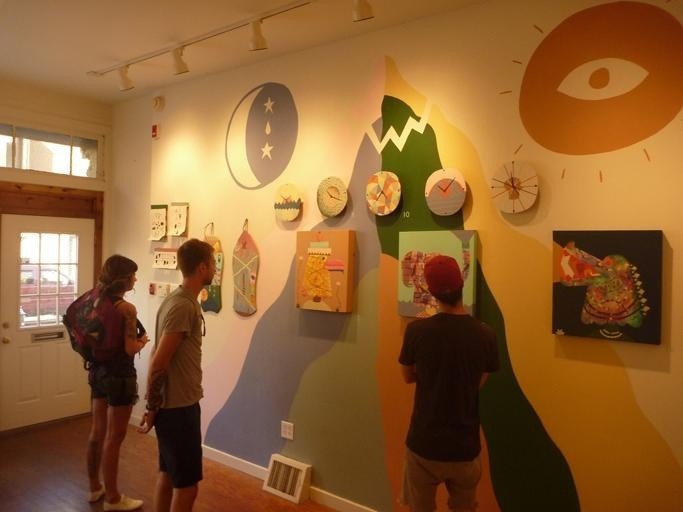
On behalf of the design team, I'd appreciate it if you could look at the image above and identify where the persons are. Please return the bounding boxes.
[398,255,499,512]
[136,238,217,512]
[62,254,151,511]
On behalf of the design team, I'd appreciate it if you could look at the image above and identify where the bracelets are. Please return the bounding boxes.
[146,403,160,411]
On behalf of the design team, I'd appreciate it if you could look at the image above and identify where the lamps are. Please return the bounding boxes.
[84,1,374,93]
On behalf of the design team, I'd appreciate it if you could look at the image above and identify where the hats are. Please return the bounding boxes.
[423,255,464,294]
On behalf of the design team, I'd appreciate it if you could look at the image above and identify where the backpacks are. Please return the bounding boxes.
[63,285,124,367]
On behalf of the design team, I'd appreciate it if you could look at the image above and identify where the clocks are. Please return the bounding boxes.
[270,159,540,225]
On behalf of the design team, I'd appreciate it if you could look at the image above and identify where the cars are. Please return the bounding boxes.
[20,264,76,317]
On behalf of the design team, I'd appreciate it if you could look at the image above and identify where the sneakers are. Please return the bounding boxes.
[87,480,106,502]
[102,493,144,511]
